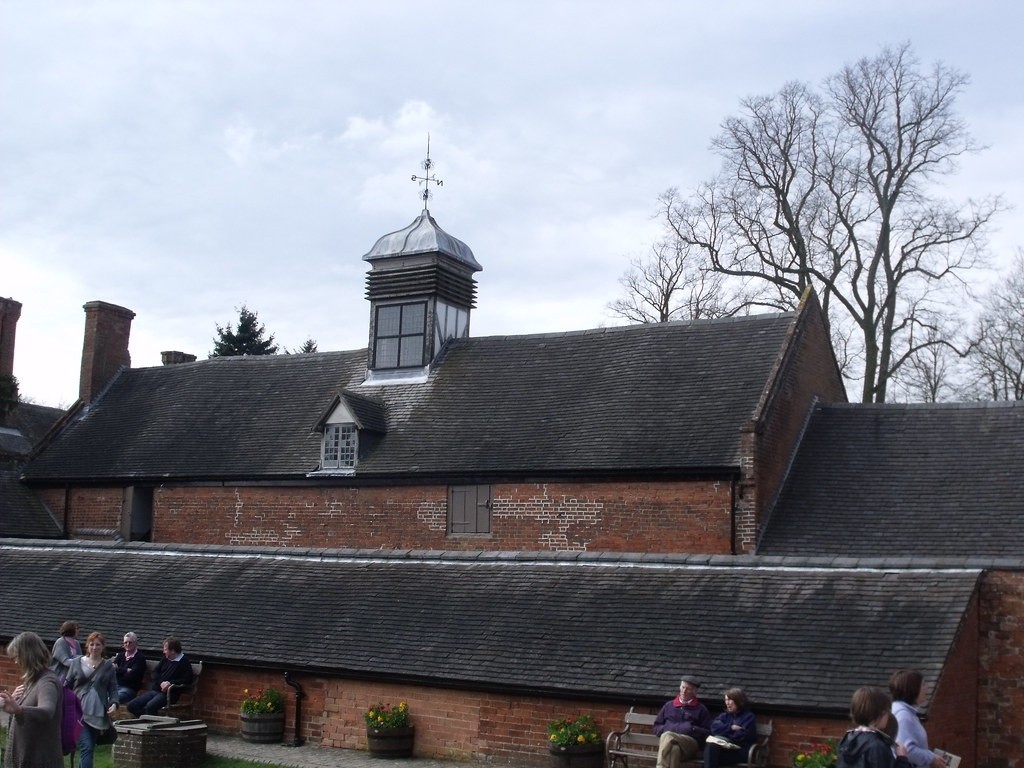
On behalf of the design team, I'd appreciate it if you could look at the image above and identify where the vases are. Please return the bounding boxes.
[366,730,414,760]
[550,742,605,768]
[242,715,286,745]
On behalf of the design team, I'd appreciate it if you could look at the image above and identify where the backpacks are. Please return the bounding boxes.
[37,673,84,756]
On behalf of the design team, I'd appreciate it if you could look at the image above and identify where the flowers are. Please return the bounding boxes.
[791,738,837,768]
[546,713,598,744]
[240,688,286,716]
[363,699,409,727]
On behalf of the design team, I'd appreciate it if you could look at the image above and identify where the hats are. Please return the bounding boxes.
[680,676,701,689]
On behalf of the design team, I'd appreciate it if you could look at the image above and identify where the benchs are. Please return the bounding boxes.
[606,707,773,768]
[146,659,203,719]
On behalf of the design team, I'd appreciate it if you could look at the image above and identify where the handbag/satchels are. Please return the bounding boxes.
[706,736,742,749]
[94,725,118,745]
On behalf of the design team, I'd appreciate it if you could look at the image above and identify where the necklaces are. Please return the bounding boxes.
[86,657,102,669]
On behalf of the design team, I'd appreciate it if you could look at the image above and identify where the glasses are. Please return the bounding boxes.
[123,642,133,644]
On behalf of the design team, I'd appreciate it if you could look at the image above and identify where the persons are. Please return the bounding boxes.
[51,619,83,688]
[652,675,713,768]
[837,685,912,768]
[888,670,950,768]
[703,686,758,768]
[0,632,65,768]
[64,631,120,768]
[127,637,193,718]
[113,631,147,704]
